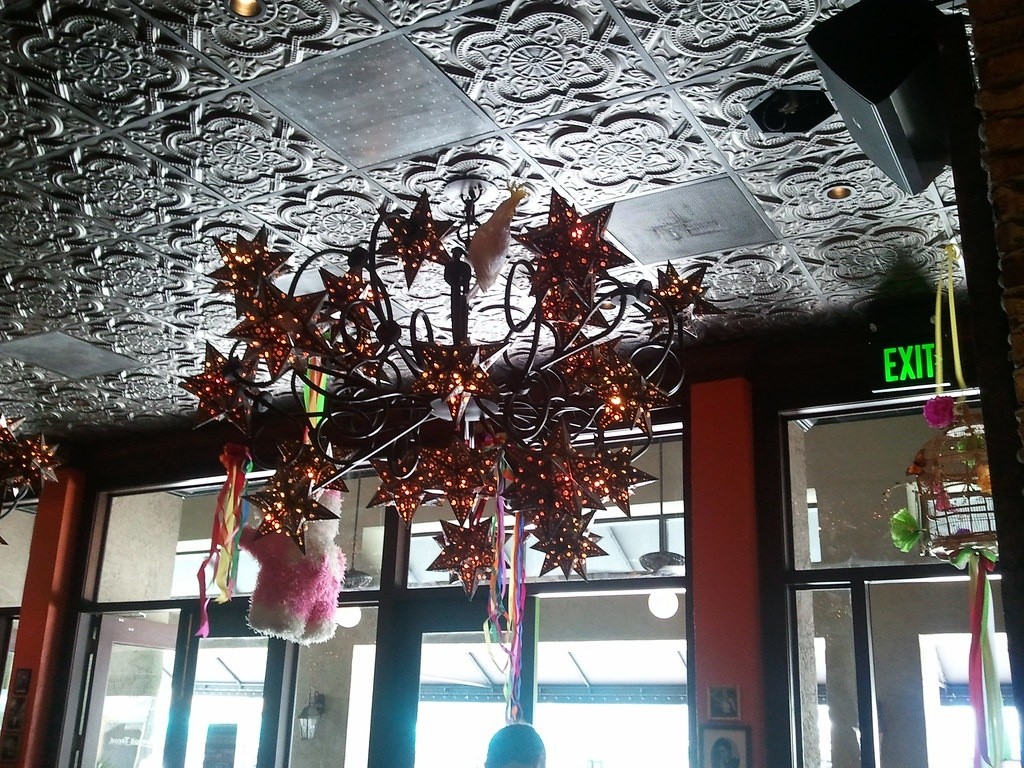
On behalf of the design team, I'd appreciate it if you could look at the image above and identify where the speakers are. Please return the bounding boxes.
[804,0,979,197]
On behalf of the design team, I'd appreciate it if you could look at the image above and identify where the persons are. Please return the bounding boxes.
[484,723,547,768]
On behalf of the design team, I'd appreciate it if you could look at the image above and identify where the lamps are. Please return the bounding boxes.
[181,174,729,601]
[0,413,63,519]
[297,686,324,741]
[639,441,686,618]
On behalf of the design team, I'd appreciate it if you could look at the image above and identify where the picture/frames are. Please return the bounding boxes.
[8,695,29,733]
[706,683,742,721]
[13,668,32,693]
[1,735,21,763]
[697,724,753,768]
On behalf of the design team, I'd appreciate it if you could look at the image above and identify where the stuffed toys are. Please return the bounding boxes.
[232,487,346,646]
